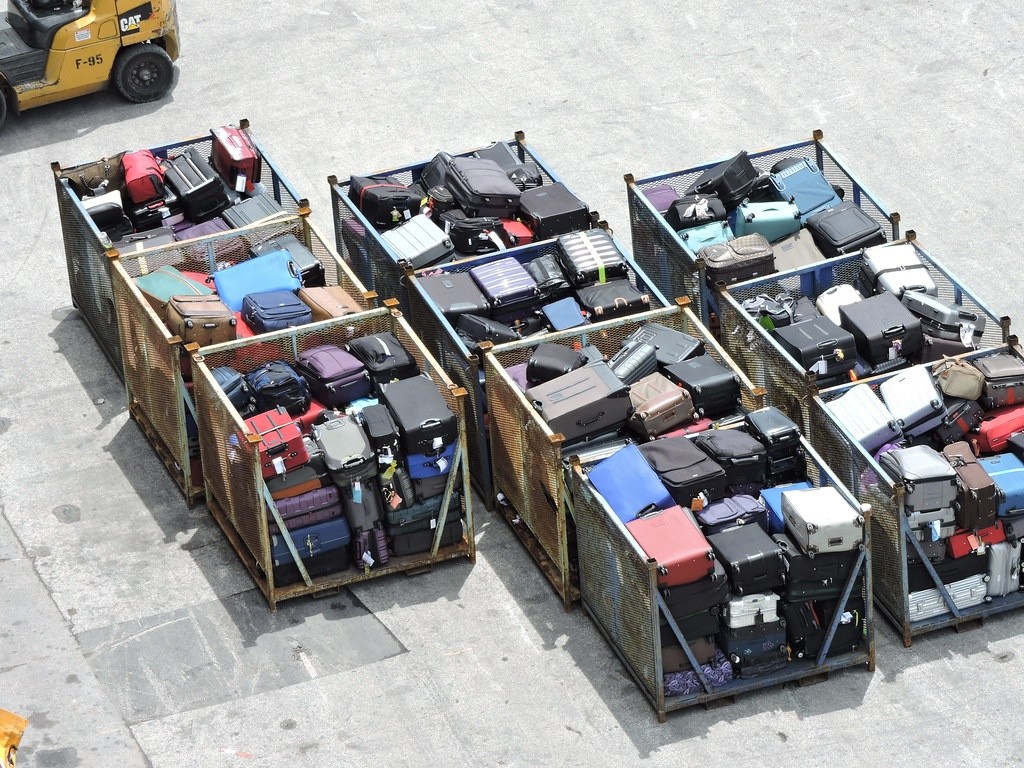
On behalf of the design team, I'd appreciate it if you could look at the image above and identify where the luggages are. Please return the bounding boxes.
[63,117,1024,702]
